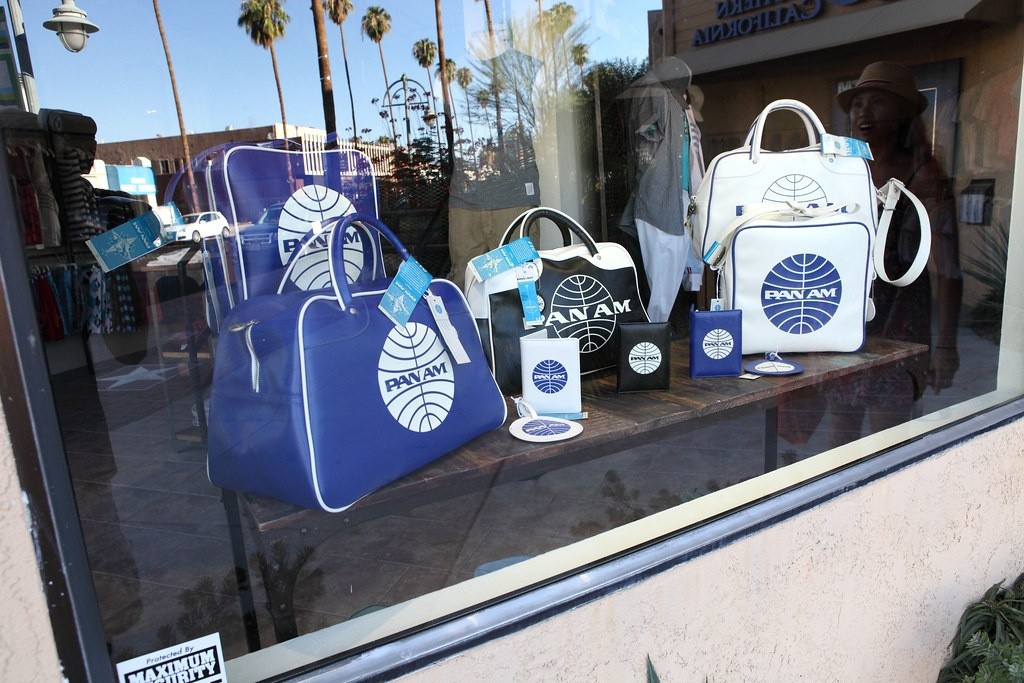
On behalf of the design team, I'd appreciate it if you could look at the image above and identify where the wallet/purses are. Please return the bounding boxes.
[616,321,671,394]
[688,303,743,378]
[519,329,583,413]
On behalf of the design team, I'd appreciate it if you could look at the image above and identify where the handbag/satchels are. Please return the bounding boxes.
[464,206,655,395]
[684,99,881,279]
[703,199,873,354]
[163,130,388,338]
[204,215,508,518]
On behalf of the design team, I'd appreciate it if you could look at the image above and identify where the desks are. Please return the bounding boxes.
[222,329,931,653]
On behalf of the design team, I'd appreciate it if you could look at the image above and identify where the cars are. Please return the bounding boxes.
[165,212,231,243]
[235,203,285,246]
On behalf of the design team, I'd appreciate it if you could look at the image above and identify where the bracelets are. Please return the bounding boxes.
[935,346,958,350]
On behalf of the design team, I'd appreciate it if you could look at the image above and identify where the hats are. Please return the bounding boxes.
[686,84,704,122]
[651,56,690,110]
[834,61,929,117]
[680,59,692,105]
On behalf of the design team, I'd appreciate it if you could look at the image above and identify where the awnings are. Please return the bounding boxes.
[628,0,1017,90]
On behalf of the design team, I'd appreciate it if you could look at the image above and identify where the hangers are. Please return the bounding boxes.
[25,245,189,276]
[3,131,129,157]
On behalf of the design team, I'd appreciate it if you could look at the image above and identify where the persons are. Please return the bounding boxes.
[432,28,547,292]
[825,59,963,451]
[618,55,712,329]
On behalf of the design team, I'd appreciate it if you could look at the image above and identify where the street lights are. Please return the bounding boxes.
[8,1,102,117]
